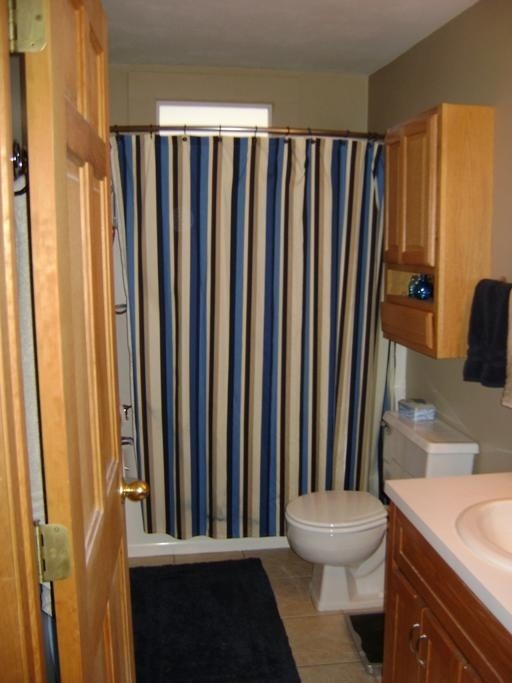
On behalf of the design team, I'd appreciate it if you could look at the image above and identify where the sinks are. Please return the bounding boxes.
[454,496,511,568]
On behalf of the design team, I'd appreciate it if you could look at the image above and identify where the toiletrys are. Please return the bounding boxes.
[408,275,418,297]
[415,273,432,300]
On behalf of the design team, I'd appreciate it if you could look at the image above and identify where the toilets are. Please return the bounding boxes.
[283,409,481,615]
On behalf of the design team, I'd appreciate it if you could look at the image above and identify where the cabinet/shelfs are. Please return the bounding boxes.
[381,478,512,682]
[378,102,498,361]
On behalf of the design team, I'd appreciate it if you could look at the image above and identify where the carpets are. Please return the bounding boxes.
[350,611,388,664]
[129,557,302,681]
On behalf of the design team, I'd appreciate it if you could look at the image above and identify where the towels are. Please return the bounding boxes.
[500,291,512,409]
[462,278,511,388]
